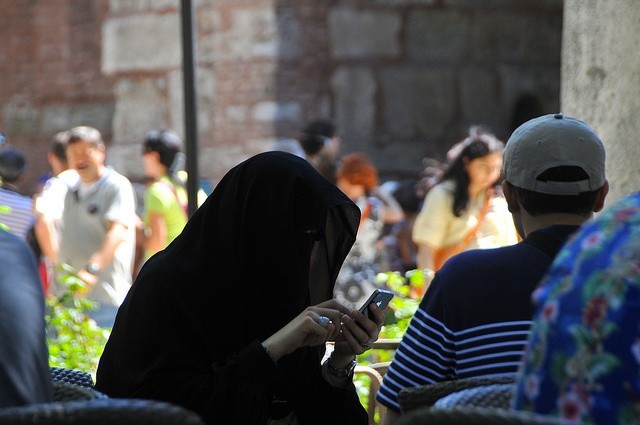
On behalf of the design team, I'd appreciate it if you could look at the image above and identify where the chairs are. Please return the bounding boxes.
[351,355,398,424]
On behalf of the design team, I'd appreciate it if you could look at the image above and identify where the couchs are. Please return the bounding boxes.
[399,189,640,424]
[0,228,200,424]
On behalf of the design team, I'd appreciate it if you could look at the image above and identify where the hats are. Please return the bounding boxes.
[503,112,606,196]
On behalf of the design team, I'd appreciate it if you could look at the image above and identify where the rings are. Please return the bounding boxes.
[361,337,373,346]
[319,315,330,329]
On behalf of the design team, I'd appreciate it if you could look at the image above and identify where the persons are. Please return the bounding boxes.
[373,111,609,425]
[35,125,78,230]
[95,148,387,425]
[294,115,342,175]
[2,144,54,264]
[509,188,640,424]
[45,125,135,344]
[328,149,406,312]
[132,213,146,249]
[133,126,209,279]
[410,132,505,289]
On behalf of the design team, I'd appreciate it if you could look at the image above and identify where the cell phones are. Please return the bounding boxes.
[352,288,394,323]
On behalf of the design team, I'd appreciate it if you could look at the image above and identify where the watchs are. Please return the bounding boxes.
[329,350,358,378]
[82,261,106,278]
